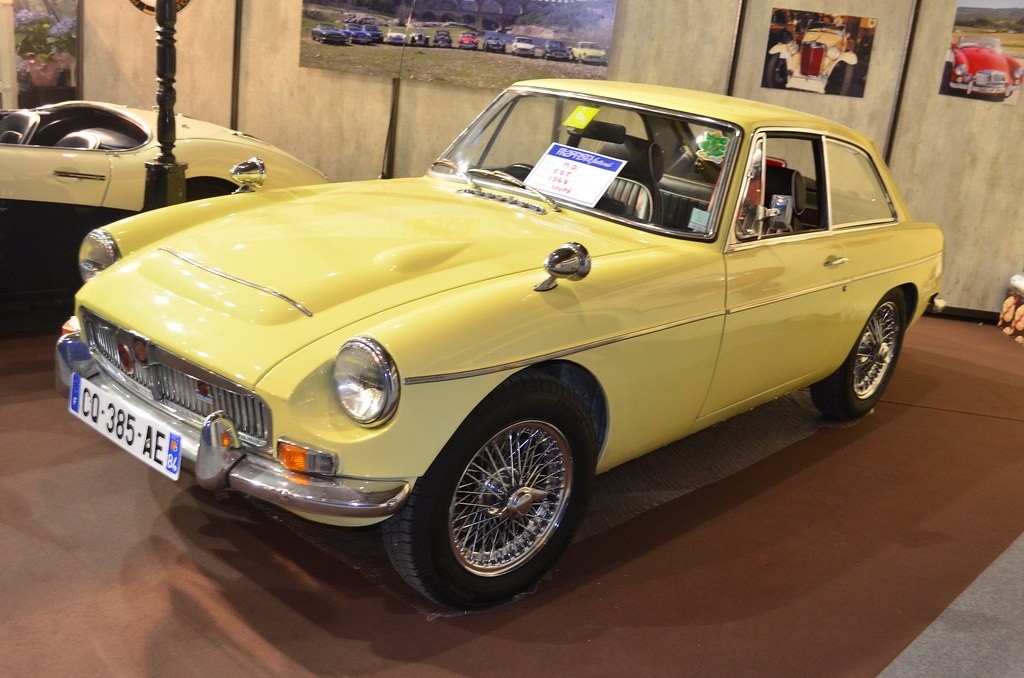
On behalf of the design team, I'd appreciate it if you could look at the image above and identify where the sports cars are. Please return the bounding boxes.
[947,35,1024,102]
[0,100,330,241]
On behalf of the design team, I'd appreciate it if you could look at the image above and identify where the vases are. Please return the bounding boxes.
[30,64,60,86]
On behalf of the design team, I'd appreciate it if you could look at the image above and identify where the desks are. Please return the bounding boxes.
[17,85,76,109]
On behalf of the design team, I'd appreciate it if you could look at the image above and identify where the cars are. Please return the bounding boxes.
[56,79,944,610]
[311,23,606,64]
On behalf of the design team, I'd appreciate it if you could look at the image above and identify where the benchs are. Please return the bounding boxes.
[659,174,715,230]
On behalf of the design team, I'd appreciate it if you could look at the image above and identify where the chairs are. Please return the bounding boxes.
[595,135,664,225]
[0,109,41,145]
[765,164,807,232]
[54,132,101,150]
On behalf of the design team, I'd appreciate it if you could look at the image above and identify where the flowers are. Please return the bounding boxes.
[14,8,79,73]
